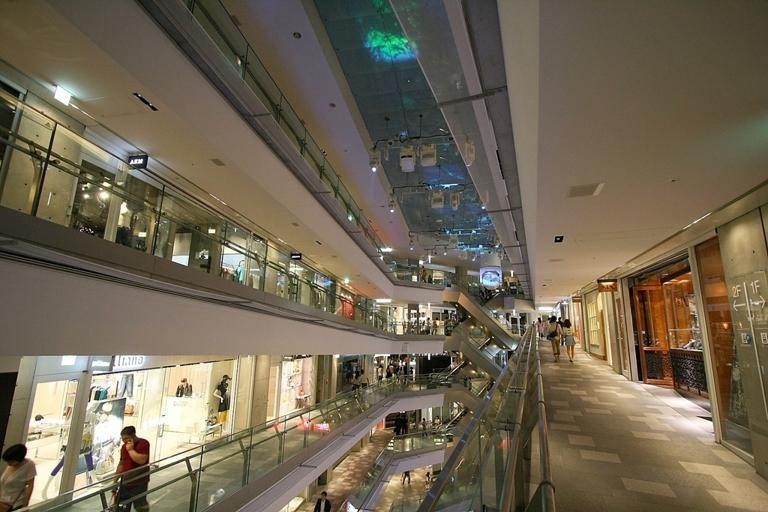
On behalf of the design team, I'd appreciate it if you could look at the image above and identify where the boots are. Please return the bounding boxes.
[552,355,559,362]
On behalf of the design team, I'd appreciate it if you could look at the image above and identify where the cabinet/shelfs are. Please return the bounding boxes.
[26,416,69,458]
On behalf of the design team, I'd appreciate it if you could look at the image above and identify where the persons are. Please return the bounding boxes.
[402,415,408,433]
[110,427,151,512]
[562,319,577,364]
[175,378,194,397]
[400,468,413,483]
[1,442,35,511]
[433,416,440,429]
[547,316,563,362]
[535,315,563,340]
[116,201,134,246]
[127,211,146,247]
[213,374,232,431]
[421,416,429,439]
[424,314,455,336]
[479,286,493,303]
[395,415,402,434]
[350,361,411,391]
[314,491,331,512]
[428,274,433,283]
[420,264,428,283]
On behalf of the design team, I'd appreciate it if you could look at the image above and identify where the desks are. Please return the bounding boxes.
[179,424,223,450]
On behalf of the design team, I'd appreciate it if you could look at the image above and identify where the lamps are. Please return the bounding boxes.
[368,115,502,257]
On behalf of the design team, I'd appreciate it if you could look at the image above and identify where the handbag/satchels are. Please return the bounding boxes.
[100,493,119,512]
[548,331,558,338]
